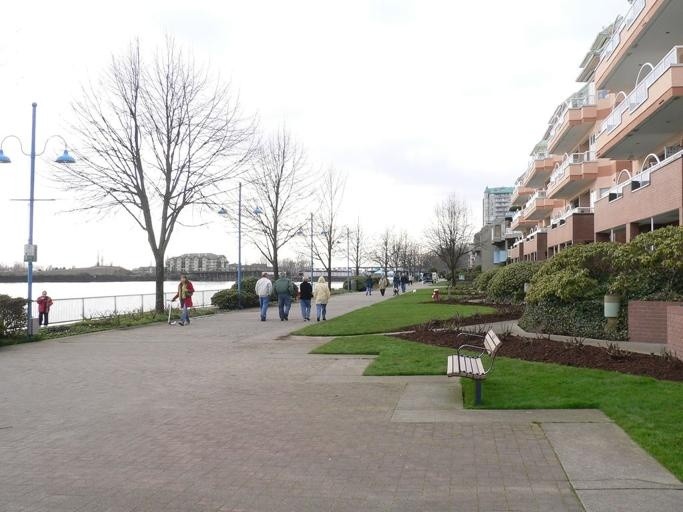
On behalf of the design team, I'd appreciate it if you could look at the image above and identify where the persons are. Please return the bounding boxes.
[272,273,294,321]
[254,273,273,321]
[379,275,388,296]
[393,270,414,293]
[314,276,330,321]
[364,275,373,296]
[37,291,53,328]
[300,276,314,322]
[170,275,195,326]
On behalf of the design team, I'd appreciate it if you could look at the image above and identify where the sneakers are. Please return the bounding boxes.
[178,320,190,326]
[281,317,288,321]
[304,318,310,321]
[261,318,266,321]
[317,319,326,321]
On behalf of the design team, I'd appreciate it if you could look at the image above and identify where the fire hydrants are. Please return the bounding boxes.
[431,288,441,303]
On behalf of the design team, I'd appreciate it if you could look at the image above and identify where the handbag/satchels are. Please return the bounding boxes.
[185,291,192,296]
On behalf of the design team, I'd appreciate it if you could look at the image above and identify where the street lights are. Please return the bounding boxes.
[217,182,264,309]
[0,102,76,337]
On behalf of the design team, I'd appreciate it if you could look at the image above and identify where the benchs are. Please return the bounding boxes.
[446,328,503,404]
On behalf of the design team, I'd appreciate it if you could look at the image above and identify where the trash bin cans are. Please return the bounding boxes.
[351,280,358,291]
